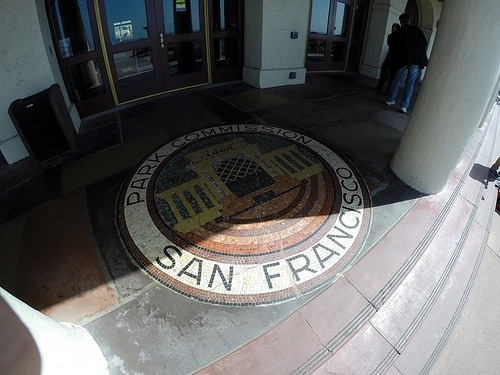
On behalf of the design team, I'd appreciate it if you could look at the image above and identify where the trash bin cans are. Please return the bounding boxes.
[6,83,80,167]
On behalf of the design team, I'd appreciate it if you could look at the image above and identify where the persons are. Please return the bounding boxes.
[385,12,429,114]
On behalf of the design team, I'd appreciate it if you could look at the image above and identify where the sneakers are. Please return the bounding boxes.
[386,100,395,105]
[400,107,407,114]
